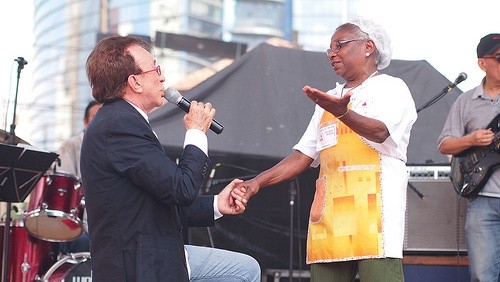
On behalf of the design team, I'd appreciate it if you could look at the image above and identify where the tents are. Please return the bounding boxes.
[148,41,464,282]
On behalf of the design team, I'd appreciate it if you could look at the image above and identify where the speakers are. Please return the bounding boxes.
[404,163,469,255]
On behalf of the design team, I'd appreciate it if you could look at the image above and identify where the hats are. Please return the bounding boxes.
[336,20,392,70]
[477,34,500,58]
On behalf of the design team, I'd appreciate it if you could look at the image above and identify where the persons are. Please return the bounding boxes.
[54,100,103,254]
[229,20,419,282]
[80,35,261,282]
[437,32,500,282]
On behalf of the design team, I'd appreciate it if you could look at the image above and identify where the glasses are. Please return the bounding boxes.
[142,65,161,75]
[483,53,500,63]
[327,37,367,60]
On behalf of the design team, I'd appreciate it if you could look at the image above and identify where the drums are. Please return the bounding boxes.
[0,218,60,282]
[38,251,93,282]
[24,170,84,243]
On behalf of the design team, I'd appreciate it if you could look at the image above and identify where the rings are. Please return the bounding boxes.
[316,99,318,102]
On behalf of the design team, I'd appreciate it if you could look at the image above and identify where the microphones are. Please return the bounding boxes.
[164,87,224,135]
[205,166,216,194]
[453,72,467,87]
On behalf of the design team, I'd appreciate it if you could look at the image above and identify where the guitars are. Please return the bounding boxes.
[450,111,500,198]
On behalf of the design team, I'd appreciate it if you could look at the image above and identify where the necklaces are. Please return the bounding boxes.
[341,70,376,97]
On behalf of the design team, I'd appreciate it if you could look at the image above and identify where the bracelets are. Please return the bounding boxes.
[336,109,349,119]
